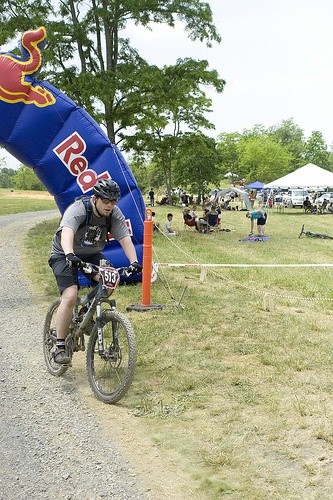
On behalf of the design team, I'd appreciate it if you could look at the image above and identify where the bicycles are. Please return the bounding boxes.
[43,260,140,405]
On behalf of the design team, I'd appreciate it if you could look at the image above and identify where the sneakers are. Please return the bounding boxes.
[85,319,96,336]
[53,347,71,364]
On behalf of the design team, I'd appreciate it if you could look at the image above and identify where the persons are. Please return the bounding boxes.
[48,179,143,364]
[163,213,178,236]
[147,187,154,207]
[152,212,160,232]
[250,191,256,208]
[303,192,333,215]
[220,191,238,211]
[246,209,267,237]
[164,186,217,206]
[183,204,221,234]
[262,192,272,208]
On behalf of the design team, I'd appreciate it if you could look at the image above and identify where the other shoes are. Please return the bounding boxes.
[257,234,264,237]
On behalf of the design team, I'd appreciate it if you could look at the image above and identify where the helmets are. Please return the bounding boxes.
[94,178,121,201]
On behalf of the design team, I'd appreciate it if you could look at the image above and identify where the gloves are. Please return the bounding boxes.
[130,261,142,274]
[66,253,81,268]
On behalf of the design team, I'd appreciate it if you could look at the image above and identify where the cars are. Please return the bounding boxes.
[309,188,333,207]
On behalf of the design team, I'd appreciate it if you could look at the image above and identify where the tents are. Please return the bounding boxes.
[244,181,271,191]
[217,187,252,210]
[263,163,333,214]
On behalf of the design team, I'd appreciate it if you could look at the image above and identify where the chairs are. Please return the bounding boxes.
[199,213,220,233]
[303,203,311,212]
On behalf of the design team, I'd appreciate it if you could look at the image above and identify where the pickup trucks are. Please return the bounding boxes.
[282,189,311,208]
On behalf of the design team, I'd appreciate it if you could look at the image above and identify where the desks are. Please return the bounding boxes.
[229,202,241,210]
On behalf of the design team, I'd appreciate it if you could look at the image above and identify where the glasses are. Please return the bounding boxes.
[100,196,116,205]
